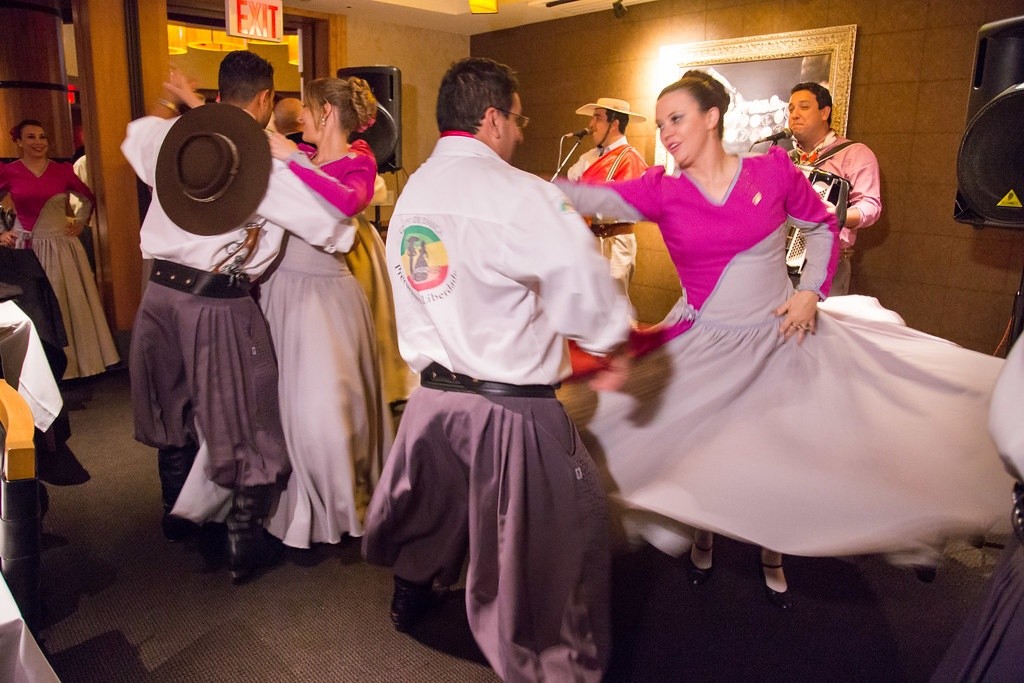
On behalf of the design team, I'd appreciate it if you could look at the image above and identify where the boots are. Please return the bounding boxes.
[157,442,197,543]
[223,482,274,584]
[390,574,425,630]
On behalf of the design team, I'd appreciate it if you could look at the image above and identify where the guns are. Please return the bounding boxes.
[213,221,262,274]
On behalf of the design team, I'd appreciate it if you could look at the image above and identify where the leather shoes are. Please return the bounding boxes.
[685,536,715,586]
[758,554,790,598]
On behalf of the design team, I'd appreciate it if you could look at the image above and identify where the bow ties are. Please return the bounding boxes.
[801,152,818,163]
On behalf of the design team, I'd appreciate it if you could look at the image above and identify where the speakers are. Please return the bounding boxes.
[337,65,403,174]
[953,15,1024,230]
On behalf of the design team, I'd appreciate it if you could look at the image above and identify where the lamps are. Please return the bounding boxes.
[186,28,249,51]
[287,34,299,65]
[247,35,289,46]
[166,23,188,56]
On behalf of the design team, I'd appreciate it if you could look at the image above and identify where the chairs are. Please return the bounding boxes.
[0,379,41,625]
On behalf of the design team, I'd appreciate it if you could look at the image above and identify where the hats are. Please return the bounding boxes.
[575,97,647,122]
[155,103,271,235]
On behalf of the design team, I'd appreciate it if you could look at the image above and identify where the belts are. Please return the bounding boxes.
[420,363,561,398]
[590,222,635,240]
[148,258,253,299]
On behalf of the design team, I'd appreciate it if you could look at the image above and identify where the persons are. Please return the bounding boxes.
[545,71,1016,613]
[933,330,1024,683]
[123,51,419,584]
[363,56,639,683]
[0,118,122,486]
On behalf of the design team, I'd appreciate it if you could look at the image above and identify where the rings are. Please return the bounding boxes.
[799,322,810,331]
[791,322,800,329]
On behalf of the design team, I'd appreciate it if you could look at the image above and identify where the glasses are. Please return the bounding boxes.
[481,106,529,130]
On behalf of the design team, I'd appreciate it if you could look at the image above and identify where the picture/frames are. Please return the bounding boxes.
[653,23,859,175]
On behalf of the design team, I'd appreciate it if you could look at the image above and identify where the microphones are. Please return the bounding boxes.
[565,128,593,138]
[754,129,793,144]
